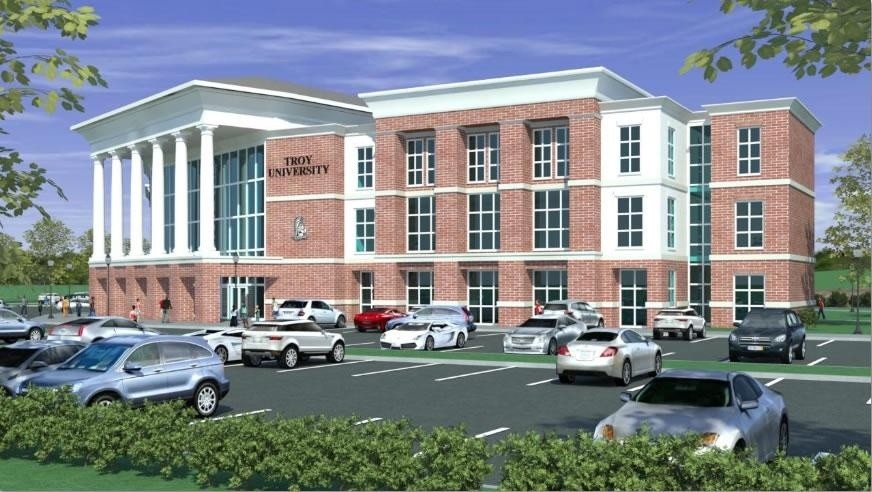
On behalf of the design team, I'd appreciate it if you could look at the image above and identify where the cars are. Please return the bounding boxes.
[592,368,790,465]
[728,308,806,364]
[182,298,477,368]
[503,299,707,386]
[0,291,230,418]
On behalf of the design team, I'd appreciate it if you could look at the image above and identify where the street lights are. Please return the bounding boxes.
[232,252,239,326]
[47,253,111,319]
